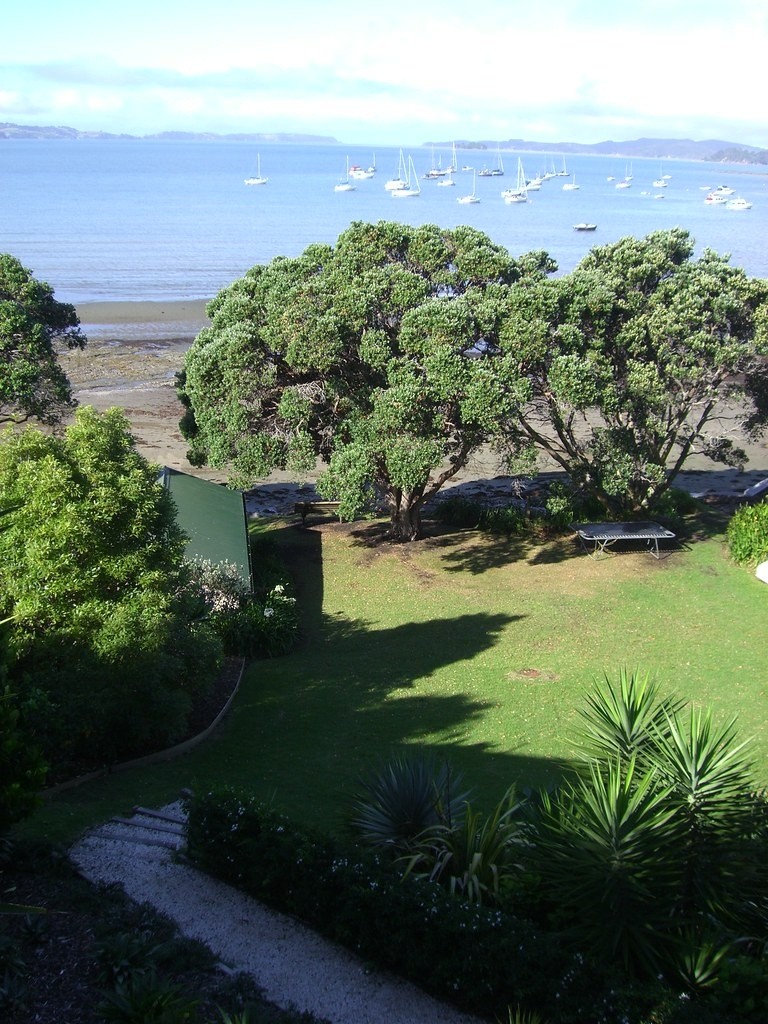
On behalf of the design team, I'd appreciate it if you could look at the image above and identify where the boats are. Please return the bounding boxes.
[725,198,752,210]
[713,185,737,195]
[573,223,598,231]
[478,168,492,176]
[699,185,712,191]
[704,193,727,205]
[461,165,474,171]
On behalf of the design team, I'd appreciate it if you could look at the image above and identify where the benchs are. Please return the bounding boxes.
[295,501,342,524]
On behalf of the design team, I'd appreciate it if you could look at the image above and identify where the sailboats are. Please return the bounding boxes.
[333,154,357,192]
[500,150,569,205]
[383,148,408,190]
[608,157,671,200]
[491,145,504,176]
[421,140,458,179]
[437,164,457,187]
[349,150,378,181]
[243,152,270,185]
[563,172,580,190]
[392,154,421,197]
[456,169,482,204]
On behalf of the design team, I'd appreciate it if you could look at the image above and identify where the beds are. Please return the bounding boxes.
[567,521,676,561]
